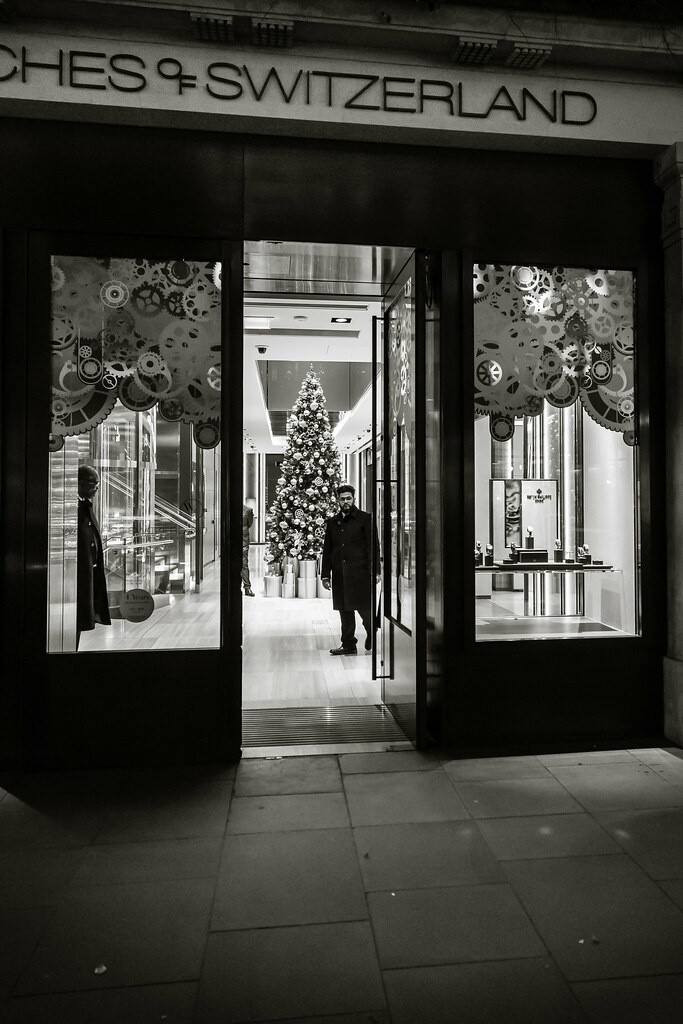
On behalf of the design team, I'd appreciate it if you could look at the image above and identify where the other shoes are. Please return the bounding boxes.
[328,646,357,655]
[245,590,255,597]
[364,633,371,650]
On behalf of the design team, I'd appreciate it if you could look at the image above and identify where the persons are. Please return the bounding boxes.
[320,486,380,655]
[76,465,110,649]
[242,505,254,597]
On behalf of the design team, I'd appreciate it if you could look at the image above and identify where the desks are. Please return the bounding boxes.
[475,566,618,617]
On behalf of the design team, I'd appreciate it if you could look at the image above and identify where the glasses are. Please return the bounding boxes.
[89,481,101,487]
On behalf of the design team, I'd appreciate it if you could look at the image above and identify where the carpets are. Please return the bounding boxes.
[241,703,408,748]
[386,703,417,747]
